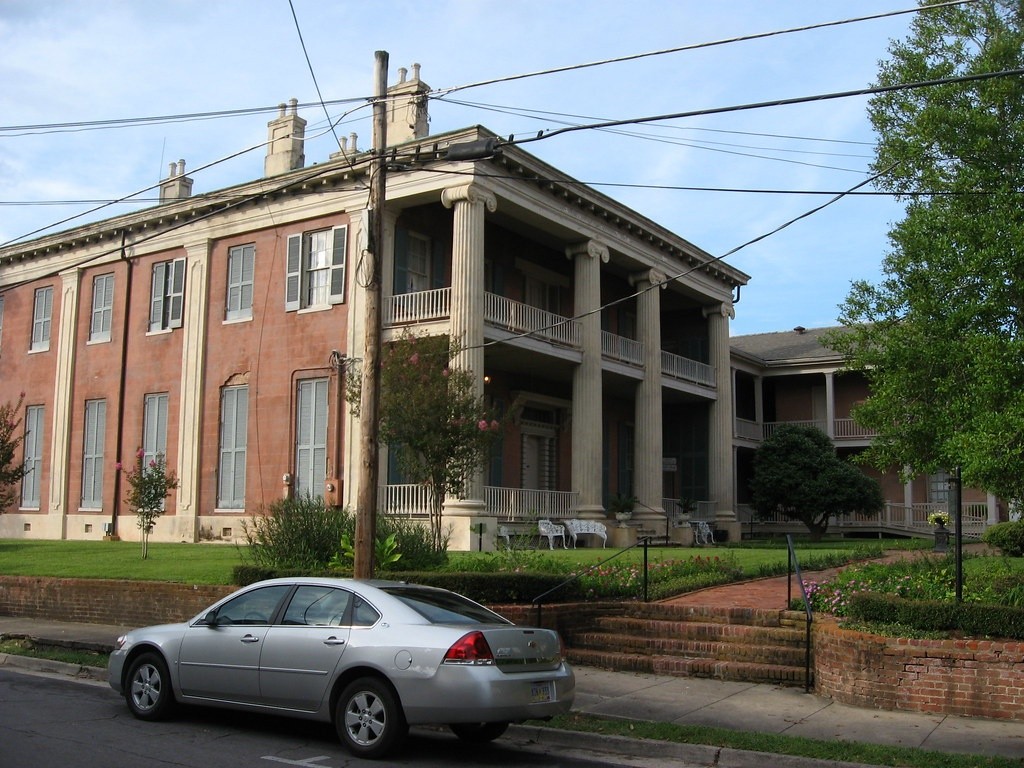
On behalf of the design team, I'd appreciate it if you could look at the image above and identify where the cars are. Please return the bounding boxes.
[106,576,577,758]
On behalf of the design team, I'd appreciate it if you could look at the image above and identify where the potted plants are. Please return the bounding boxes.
[674,497,698,528]
[605,490,634,529]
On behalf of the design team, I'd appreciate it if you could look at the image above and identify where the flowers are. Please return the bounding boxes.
[927,510,953,527]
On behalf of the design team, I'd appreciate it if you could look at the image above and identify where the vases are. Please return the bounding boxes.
[935,518,945,531]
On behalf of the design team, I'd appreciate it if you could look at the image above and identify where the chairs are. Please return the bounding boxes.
[699,521,716,544]
[288,592,321,622]
[497,525,511,552]
[538,519,568,551]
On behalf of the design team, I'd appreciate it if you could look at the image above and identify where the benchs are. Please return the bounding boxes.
[564,518,608,550]
